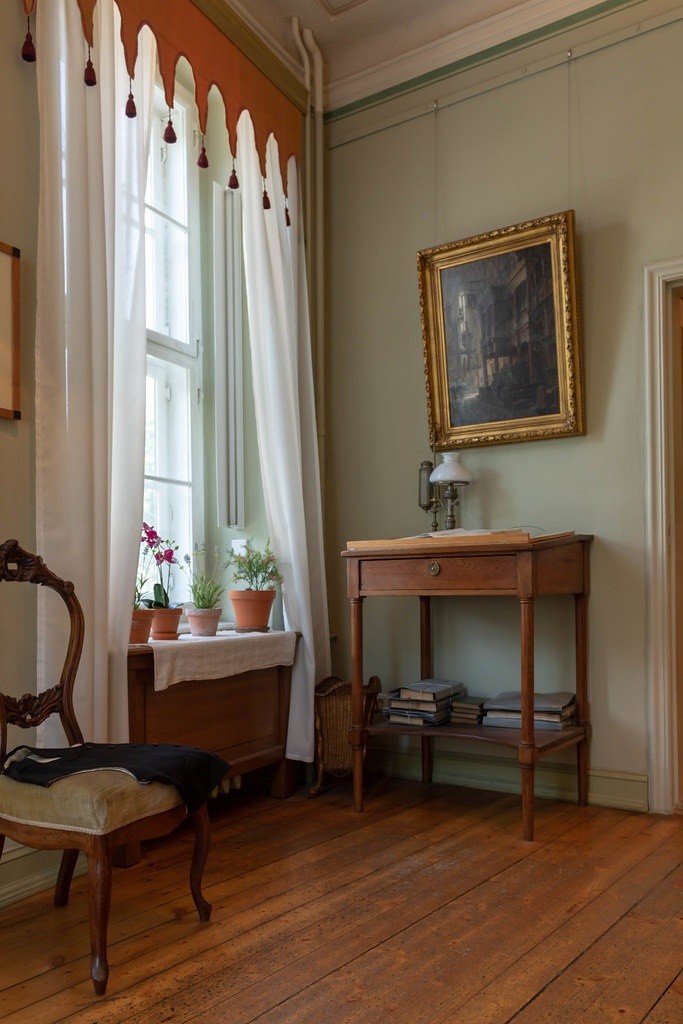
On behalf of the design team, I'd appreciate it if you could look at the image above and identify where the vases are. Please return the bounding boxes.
[185,606,225,637]
[148,607,184,640]
[130,608,154,644]
[228,589,280,631]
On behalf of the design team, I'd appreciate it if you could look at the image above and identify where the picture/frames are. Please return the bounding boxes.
[414,207,590,455]
[0,240,24,422]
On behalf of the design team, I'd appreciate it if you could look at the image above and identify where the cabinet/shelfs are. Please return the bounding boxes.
[340,531,598,842]
[117,631,302,802]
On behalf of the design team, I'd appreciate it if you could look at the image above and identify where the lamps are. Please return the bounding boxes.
[415,453,472,533]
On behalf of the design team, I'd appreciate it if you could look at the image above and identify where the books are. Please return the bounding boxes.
[393,528,524,538]
[484,692,577,731]
[377,679,464,726]
[450,696,491,725]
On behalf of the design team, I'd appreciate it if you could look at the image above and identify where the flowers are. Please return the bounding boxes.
[129,520,286,609]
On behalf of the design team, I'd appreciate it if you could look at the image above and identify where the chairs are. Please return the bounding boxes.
[0,537,214,994]
